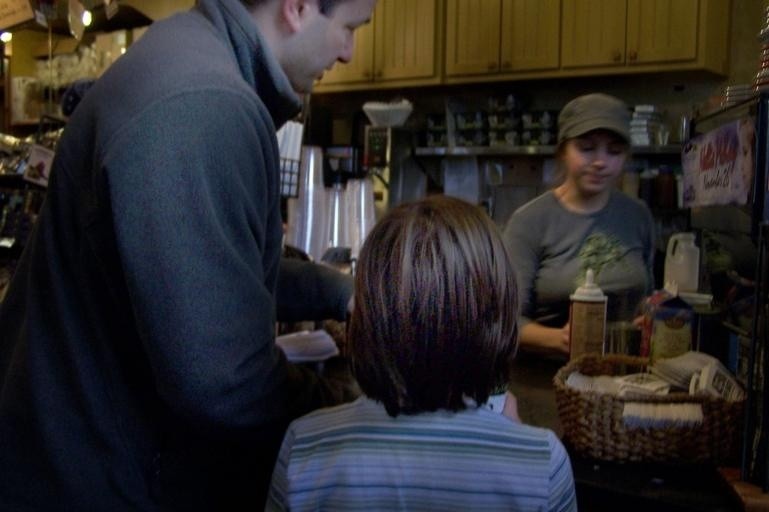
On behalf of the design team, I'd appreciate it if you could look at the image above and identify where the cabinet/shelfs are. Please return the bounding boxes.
[679,87,769,512]
[314,1,729,93]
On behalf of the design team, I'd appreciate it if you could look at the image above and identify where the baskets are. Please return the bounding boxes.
[553,353,749,465]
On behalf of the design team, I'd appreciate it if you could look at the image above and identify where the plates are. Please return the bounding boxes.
[276,329,340,363]
[678,292,713,306]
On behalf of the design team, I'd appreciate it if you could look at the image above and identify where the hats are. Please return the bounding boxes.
[558,93,633,146]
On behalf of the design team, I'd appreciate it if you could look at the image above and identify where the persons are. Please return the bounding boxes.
[2,0,380,510]
[504,93,689,370]
[274,198,363,410]
[262,191,580,510]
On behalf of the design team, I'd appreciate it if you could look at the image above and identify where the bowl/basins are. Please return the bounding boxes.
[362,101,413,128]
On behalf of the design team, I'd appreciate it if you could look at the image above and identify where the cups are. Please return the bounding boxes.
[284,145,376,266]
[656,123,672,145]
[462,386,509,414]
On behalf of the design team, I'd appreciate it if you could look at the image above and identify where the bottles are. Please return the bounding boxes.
[566,267,608,365]
[678,112,690,142]
